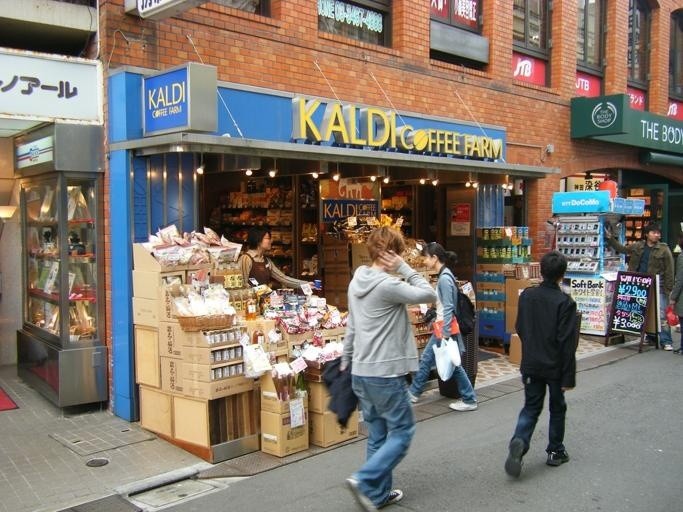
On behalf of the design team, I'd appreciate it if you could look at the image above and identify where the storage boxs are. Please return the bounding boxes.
[260,372,359,458]
[505,306,522,364]
[323,247,373,314]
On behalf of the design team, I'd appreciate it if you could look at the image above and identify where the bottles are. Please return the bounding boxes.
[482,224,530,258]
[245,291,256,319]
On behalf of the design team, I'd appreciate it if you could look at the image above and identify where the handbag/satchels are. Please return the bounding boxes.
[439,273,475,335]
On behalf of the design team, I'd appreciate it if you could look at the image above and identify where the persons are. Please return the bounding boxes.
[669,232,683,356]
[339,224,439,511]
[408,242,478,412]
[504,249,578,478]
[238,225,323,292]
[604,222,676,352]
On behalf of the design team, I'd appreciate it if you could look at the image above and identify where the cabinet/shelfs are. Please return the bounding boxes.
[380,182,436,242]
[213,175,324,298]
[553,217,627,336]
[474,229,532,344]
[16,172,109,408]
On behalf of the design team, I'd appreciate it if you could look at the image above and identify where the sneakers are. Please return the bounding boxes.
[345,473,403,512]
[546,449,569,466]
[449,399,477,411]
[505,435,528,477]
[663,344,674,351]
[406,388,419,403]
[643,338,657,346]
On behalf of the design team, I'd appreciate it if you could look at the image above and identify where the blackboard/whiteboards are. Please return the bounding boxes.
[608,271,653,336]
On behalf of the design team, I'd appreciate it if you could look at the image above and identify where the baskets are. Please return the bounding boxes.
[437,314,478,398]
[176,313,235,332]
